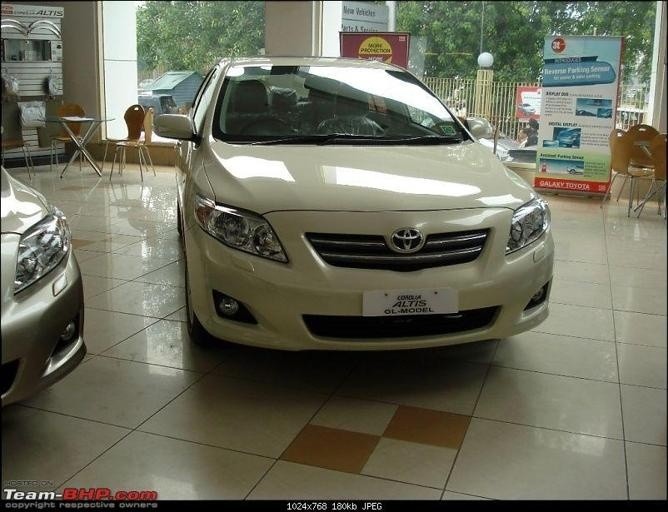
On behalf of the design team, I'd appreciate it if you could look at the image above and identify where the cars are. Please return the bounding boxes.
[464,115,523,162]
[153,54,559,351]
[1,155,88,411]
[567,165,584,175]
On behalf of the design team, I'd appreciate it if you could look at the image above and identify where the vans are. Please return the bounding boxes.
[517,103,536,115]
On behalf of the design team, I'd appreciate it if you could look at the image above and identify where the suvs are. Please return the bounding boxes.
[557,128,581,148]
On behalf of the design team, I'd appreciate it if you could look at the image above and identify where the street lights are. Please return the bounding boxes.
[475,53,494,122]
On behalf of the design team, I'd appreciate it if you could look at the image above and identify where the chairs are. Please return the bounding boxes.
[1,100,40,180]
[223,77,386,142]
[96,103,149,175]
[107,178,152,236]
[109,107,159,184]
[48,101,88,172]
[601,123,668,223]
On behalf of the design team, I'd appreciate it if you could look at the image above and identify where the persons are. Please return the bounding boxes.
[517,119,540,150]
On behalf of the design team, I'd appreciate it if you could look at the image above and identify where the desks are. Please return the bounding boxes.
[34,116,118,180]
